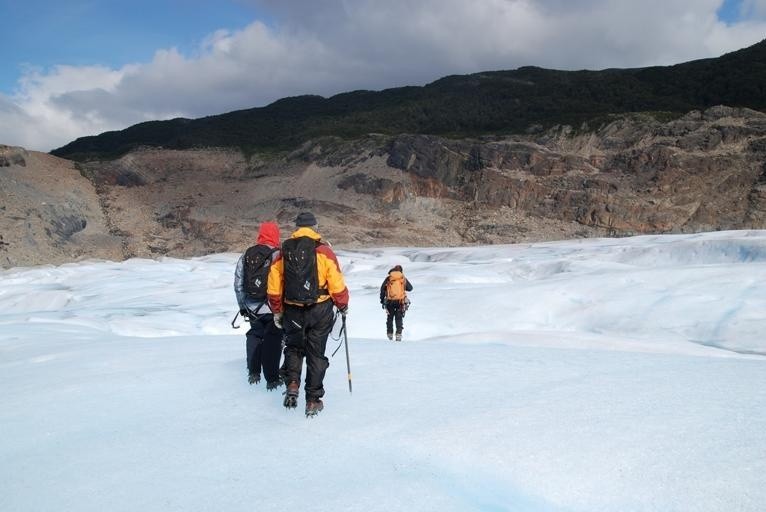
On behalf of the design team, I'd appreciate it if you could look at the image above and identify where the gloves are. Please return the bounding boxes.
[272,311,286,331]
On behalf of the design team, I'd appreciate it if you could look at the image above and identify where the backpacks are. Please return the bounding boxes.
[279,235,326,307]
[241,243,280,303]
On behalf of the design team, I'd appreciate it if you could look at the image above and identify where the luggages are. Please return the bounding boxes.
[385,270,407,303]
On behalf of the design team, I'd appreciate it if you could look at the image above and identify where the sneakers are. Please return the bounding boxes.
[395,333,402,341]
[265,376,285,391]
[304,399,324,416]
[386,333,394,341]
[248,370,262,385]
[281,381,300,409]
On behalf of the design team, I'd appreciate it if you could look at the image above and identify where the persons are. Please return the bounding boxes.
[380,265,413,342]
[232,220,285,389]
[265,213,350,417]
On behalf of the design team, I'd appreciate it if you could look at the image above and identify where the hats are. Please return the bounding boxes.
[295,212,318,228]
[394,264,403,272]
